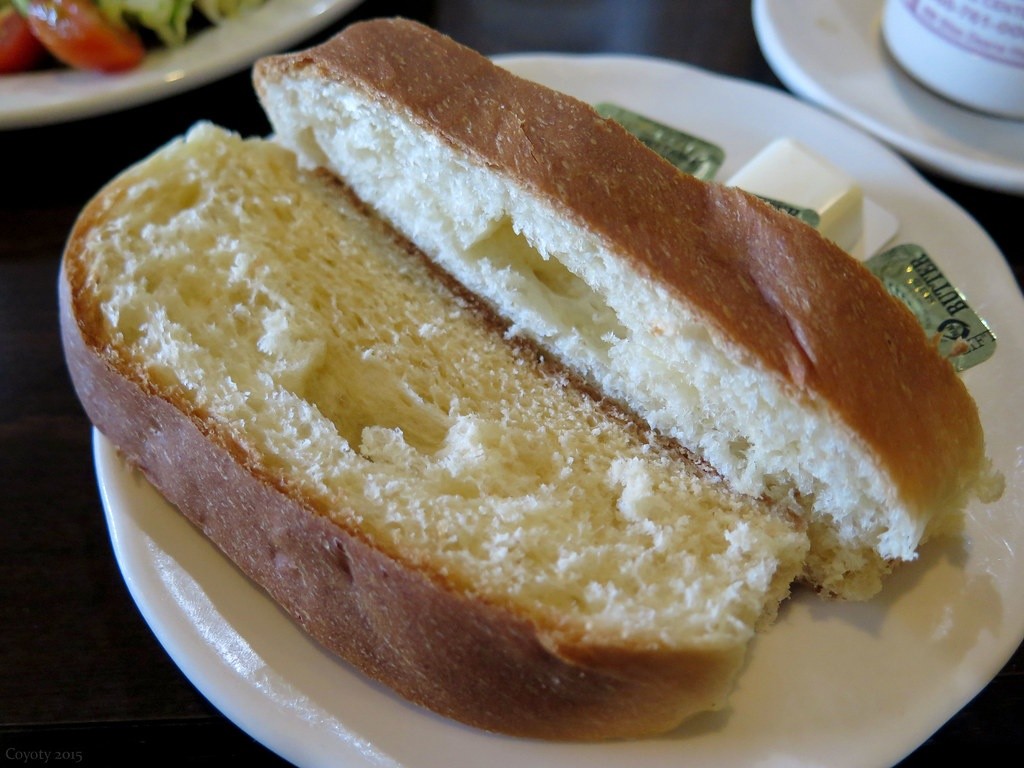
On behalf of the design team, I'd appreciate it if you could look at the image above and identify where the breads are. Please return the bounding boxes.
[56,20,979,739]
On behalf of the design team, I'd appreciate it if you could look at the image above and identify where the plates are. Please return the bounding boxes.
[0,0,363,129]
[92,53,1024,768]
[752,0,1024,192]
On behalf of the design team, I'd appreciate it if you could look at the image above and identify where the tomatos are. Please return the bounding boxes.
[0,1,143,75]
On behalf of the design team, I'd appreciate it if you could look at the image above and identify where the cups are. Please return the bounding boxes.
[879,0,1024,118]
